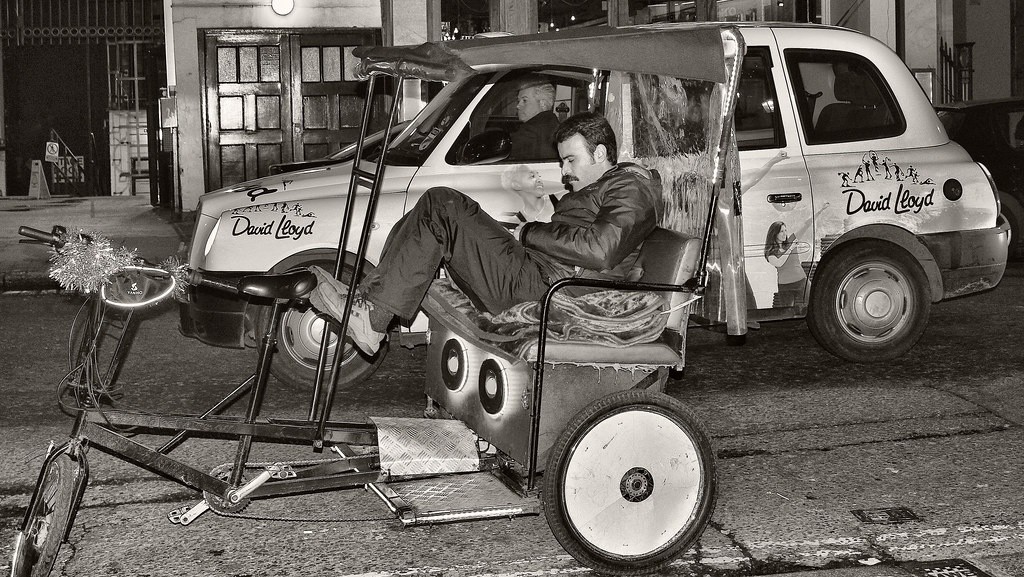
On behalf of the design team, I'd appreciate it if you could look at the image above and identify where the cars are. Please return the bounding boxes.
[268,116,525,179]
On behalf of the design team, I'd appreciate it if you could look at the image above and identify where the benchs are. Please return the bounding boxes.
[425,225,700,366]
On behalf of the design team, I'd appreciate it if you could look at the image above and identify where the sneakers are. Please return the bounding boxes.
[309,265,362,297]
[317,280,389,357]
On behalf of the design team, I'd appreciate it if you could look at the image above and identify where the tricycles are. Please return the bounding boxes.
[8,27,746,577]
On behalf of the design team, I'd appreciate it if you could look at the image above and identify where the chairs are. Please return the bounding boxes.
[815,72,890,133]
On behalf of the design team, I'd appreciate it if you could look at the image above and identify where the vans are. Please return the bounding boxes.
[173,21,1013,394]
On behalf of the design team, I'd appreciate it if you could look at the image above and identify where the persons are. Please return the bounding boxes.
[764,201,831,307]
[501,164,570,224]
[504,80,561,159]
[307,111,664,358]
